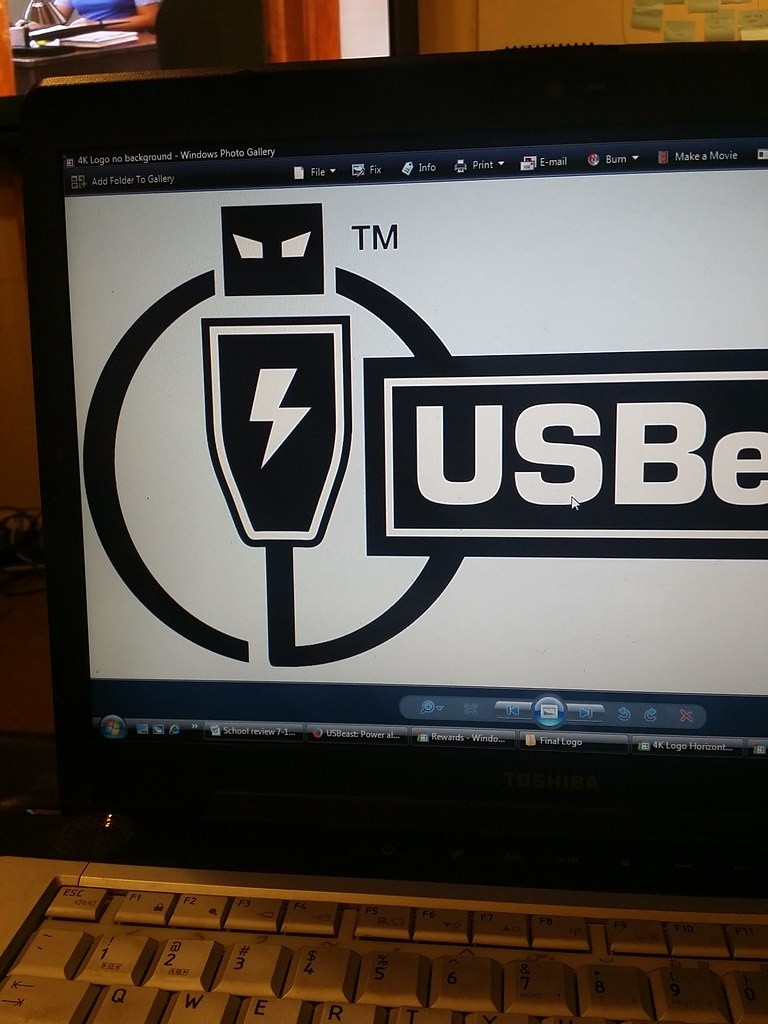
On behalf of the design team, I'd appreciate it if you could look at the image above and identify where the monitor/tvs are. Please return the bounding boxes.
[0,41,768,897]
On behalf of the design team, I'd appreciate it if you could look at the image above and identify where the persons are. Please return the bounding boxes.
[15,0,161,30]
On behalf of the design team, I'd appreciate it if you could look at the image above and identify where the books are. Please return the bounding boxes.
[29,25,138,48]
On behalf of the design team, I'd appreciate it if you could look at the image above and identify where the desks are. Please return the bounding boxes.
[12,33,158,96]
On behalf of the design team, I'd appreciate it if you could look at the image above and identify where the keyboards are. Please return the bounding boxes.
[0,855,768,1024]
